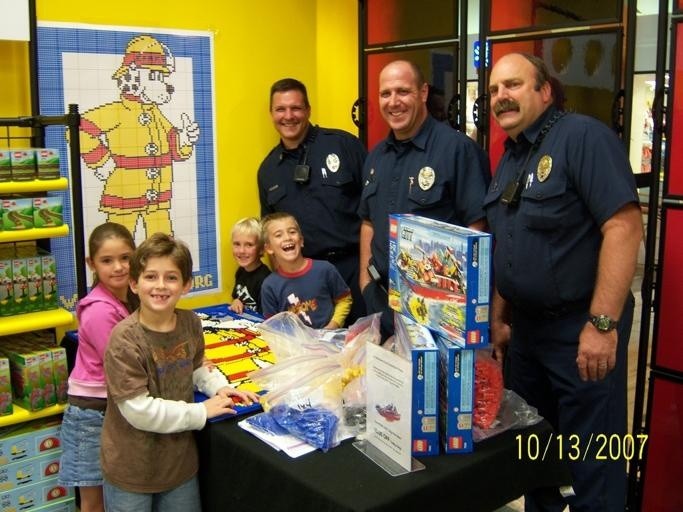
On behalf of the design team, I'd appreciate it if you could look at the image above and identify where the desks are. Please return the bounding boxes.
[65,304,555,510]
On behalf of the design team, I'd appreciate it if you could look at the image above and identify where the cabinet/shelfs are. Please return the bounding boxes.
[0,174,79,431]
